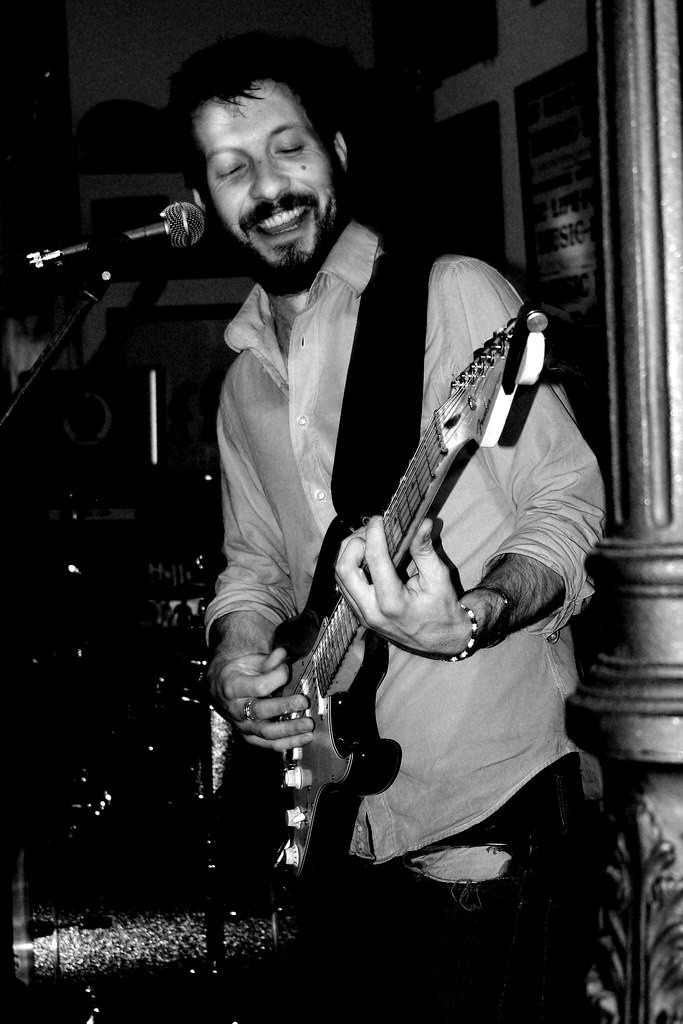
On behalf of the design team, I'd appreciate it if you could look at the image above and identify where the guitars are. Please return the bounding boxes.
[195,303,547,892]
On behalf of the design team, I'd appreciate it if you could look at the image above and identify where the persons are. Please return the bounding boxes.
[164,32,604,1024]
[160,600,172,626]
[173,601,195,630]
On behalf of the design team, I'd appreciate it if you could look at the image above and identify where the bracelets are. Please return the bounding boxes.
[464,586,511,646]
[442,604,479,663]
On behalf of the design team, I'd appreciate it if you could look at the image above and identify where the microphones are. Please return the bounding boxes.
[24,201,205,270]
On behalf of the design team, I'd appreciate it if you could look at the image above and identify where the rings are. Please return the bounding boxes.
[243,699,253,720]
